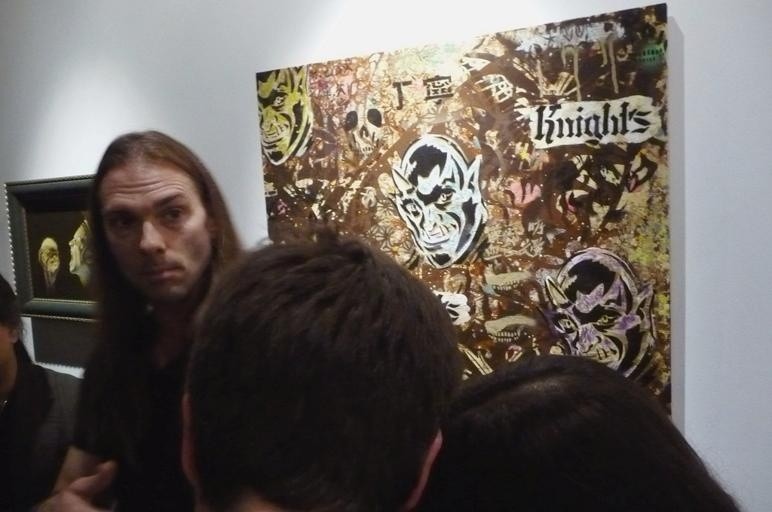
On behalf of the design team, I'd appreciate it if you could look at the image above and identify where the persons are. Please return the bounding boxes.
[418,353,742,511]
[68,218,102,287]
[179,218,463,512]
[0,273,85,512]
[36,129,244,512]
[34,237,85,300]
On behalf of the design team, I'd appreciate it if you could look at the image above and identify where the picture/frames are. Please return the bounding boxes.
[4,174,131,326]
